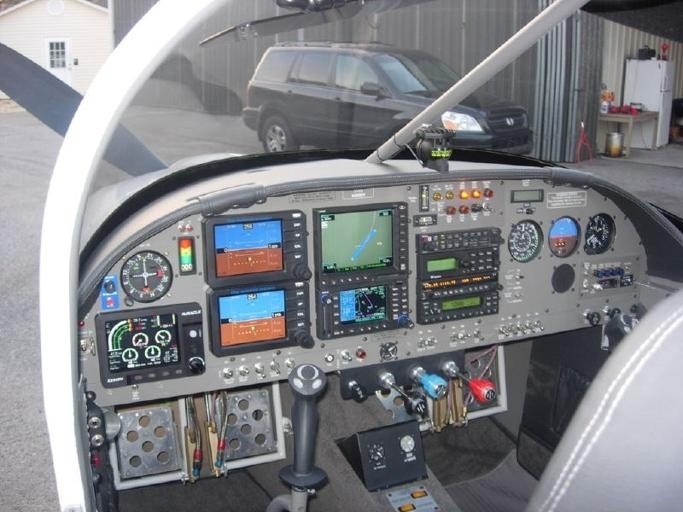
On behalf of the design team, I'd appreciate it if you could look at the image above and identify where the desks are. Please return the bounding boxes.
[599,111,659,159]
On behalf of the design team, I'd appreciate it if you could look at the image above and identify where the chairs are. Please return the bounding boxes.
[526,289,683,512]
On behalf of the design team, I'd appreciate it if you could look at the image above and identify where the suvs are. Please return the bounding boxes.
[242,41,533,155]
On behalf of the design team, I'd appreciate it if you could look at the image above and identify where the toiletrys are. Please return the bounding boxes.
[600,100,609,114]
